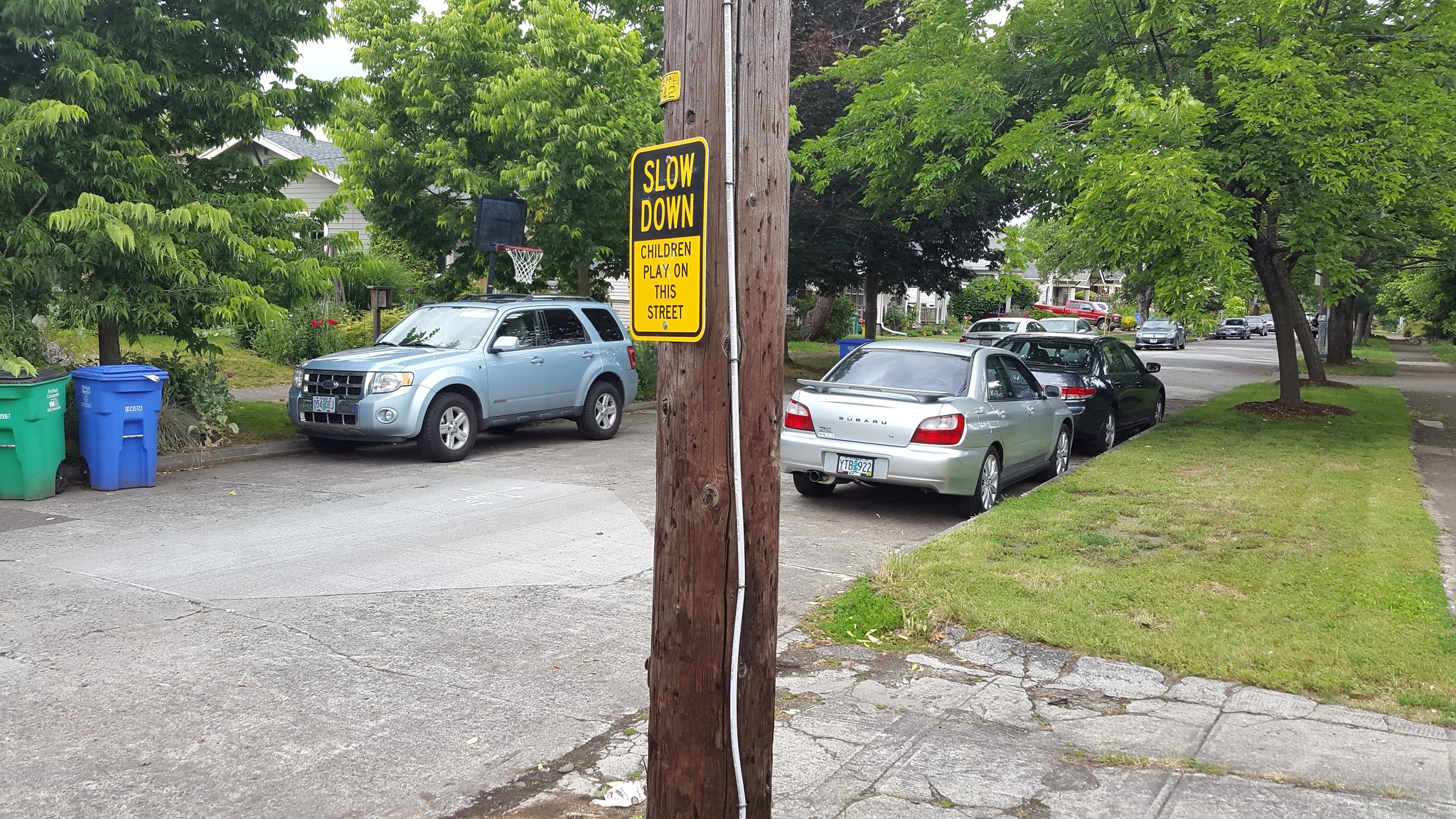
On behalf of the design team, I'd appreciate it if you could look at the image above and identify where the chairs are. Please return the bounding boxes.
[1062,353,1085,367]
[1030,349,1049,363]
[981,327,990,331]
[993,325,1001,331]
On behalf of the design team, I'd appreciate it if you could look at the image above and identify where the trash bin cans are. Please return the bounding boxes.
[1134,313,1139,324]
[69,364,170,492]
[854,313,861,334]
[835,338,876,361]
[0,369,72,501]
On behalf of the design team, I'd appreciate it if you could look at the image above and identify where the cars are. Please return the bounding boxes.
[1135,318,1186,351]
[1260,314,1275,332]
[781,340,1075,518]
[1216,318,1251,339]
[1037,318,1098,335]
[952,331,1165,458]
[1243,316,1268,336]
[960,316,1047,347]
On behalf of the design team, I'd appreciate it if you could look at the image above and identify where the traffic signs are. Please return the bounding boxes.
[629,136,706,342]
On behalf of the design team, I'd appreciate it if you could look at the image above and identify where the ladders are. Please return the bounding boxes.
[325,234,349,319]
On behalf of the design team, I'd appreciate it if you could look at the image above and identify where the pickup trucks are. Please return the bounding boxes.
[1310,312,1327,338]
[1034,300,1122,331]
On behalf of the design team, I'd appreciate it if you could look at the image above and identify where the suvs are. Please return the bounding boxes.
[286,293,638,463]
[1085,302,1113,314]
